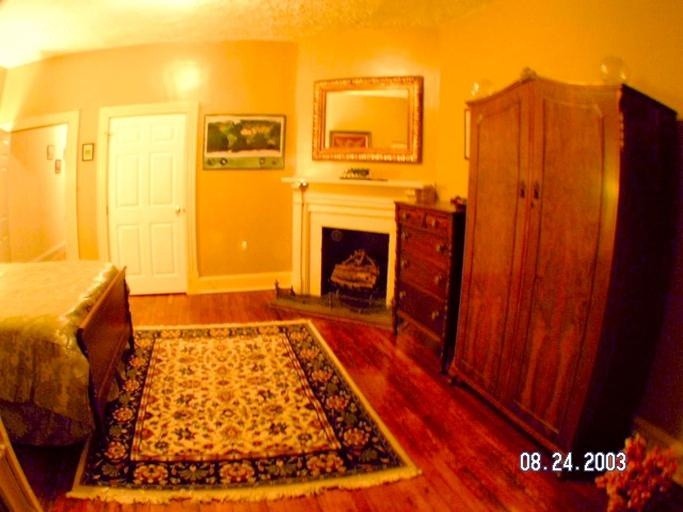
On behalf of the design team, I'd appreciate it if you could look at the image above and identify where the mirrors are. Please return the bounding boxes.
[312,76,424,164]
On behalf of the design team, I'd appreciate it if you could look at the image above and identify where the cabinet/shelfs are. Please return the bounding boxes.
[390,198,465,374]
[447,69,680,483]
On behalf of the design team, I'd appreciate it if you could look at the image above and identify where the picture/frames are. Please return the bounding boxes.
[204,113,286,169]
[82,143,94,161]
[329,130,370,149]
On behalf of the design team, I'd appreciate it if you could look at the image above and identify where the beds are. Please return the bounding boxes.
[0,256,136,449]
[64,318,423,504]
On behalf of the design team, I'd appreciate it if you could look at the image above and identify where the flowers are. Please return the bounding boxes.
[592,430,677,512]
